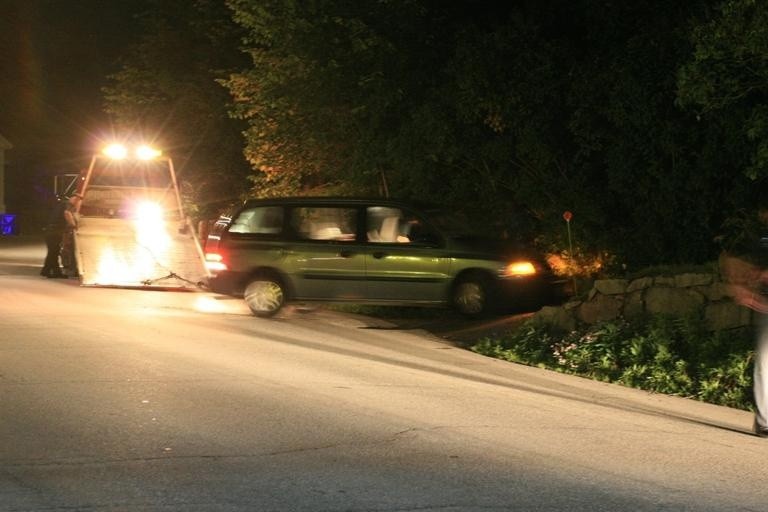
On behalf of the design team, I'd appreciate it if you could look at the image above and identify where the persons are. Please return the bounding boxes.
[40,193,84,278]
[718,244,767,435]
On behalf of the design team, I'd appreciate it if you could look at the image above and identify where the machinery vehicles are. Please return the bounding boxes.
[53,145,205,291]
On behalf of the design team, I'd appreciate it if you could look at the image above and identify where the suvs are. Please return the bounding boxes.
[206,194,538,317]
